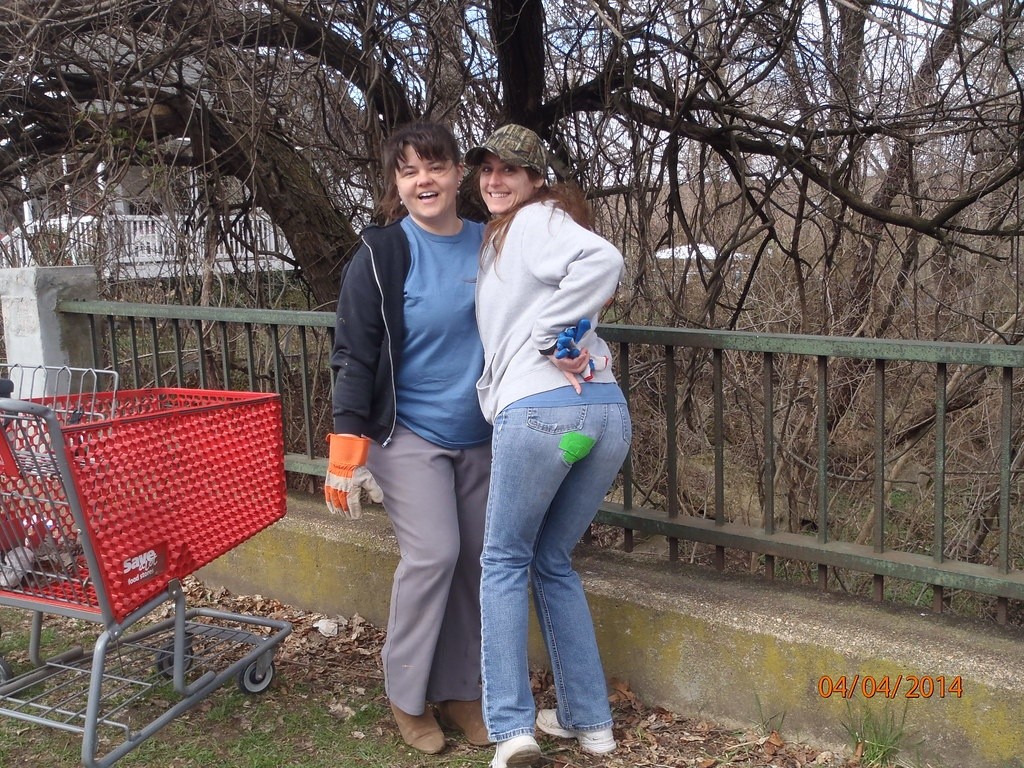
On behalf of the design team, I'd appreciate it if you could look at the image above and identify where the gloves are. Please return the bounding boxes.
[320,432,386,520]
[556,319,608,384]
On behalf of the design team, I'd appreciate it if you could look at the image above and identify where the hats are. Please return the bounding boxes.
[465,124,548,177]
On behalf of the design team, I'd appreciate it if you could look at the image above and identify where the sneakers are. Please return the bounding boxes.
[490,736,541,768]
[536,708,618,754]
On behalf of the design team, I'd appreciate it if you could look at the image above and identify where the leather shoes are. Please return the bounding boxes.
[448,701,492,746]
[392,698,446,754]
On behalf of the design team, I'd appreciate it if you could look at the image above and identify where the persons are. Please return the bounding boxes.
[463,123,632,768]
[323,124,494,755]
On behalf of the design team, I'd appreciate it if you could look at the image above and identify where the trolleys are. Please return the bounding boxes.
[0,364,295,768]
